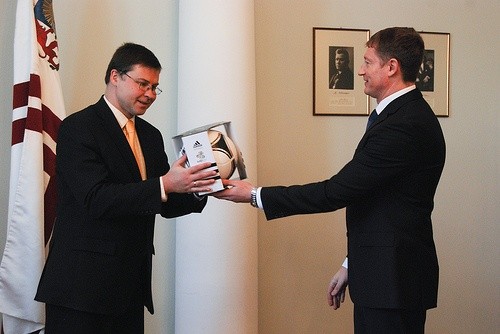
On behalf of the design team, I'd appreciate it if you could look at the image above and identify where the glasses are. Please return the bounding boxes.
[116,68,162,96]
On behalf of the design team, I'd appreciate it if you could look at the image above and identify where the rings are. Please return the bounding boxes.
[193,181,197,187]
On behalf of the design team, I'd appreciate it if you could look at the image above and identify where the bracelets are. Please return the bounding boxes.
[249,186,259,209]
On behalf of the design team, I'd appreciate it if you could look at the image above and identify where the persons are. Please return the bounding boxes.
[43,41,247,334]
[329,49,354,90]
[209,25,446,334]
[415,62,432,91]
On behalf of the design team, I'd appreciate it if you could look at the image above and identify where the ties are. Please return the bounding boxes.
[329,71,339,89]
[365,109,378,132]
[125,121,147,182]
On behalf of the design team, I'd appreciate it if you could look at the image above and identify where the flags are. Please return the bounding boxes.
[0,0,66,334]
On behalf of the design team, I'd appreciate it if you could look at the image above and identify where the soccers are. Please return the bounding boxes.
[178,129,239,180]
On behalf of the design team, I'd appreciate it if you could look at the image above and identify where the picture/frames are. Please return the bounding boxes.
[312,27,370,116]
[416,32,450,117]
